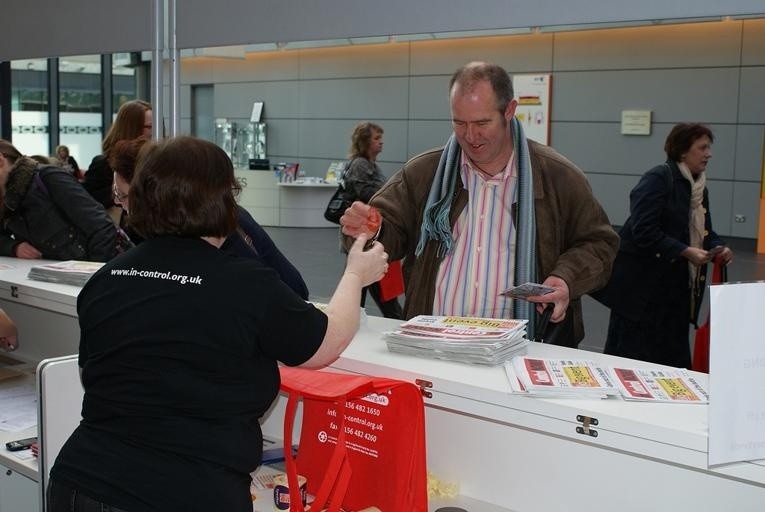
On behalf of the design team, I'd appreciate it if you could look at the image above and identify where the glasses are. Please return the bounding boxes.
[113,190,129,204]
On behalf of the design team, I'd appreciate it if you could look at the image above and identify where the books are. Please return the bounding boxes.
[381,313,531,367]
[609,368,709,405]
[504,355,621,400]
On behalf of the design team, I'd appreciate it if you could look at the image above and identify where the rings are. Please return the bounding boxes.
[384,264,390,274]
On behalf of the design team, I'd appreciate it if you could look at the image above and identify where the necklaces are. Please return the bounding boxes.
[468,157,508,180]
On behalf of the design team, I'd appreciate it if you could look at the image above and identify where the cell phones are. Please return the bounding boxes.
[5,436,40,452]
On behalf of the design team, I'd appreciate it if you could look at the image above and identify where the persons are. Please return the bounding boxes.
[324,122,405,320]
[0,91,154,266]
[603,118,735,373]
[338,58,622,351]
[0,306,19,355]
[44,134,396,511]
[108,134,312,306]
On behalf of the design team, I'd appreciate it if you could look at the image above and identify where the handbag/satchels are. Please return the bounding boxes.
[583,231,665,326]
[275,366,429,511]
[324,184,357,226]
[42,220,137,262]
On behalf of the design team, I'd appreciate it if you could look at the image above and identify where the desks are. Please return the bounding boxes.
[249,438,512,512]
[0,349,40,483]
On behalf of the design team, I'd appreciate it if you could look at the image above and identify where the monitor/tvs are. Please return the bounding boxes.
[248,158,270,170]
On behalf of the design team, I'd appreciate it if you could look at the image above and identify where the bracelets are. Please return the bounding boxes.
[374,215,384,239]
[342,269,366,287]
